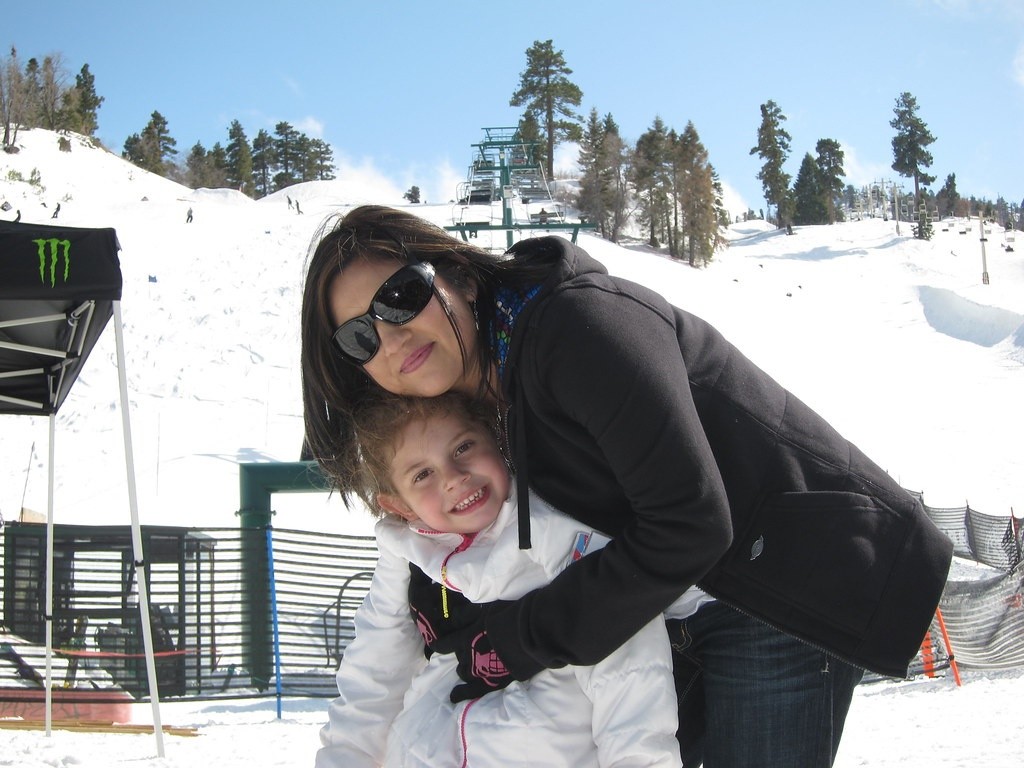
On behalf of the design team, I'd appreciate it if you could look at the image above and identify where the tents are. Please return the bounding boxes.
[0,219,165,759]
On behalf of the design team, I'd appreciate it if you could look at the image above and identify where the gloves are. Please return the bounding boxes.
[406,558,481,662]
[433,617,516,705]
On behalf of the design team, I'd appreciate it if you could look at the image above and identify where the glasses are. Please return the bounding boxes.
[331,262,438,367]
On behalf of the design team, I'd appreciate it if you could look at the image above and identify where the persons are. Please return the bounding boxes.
[315,388,684,768]
[186,208,193,223]
[300,203,955,768]
[13,210,21,222]
[51,202,61,218]
[287,196,304,214]
